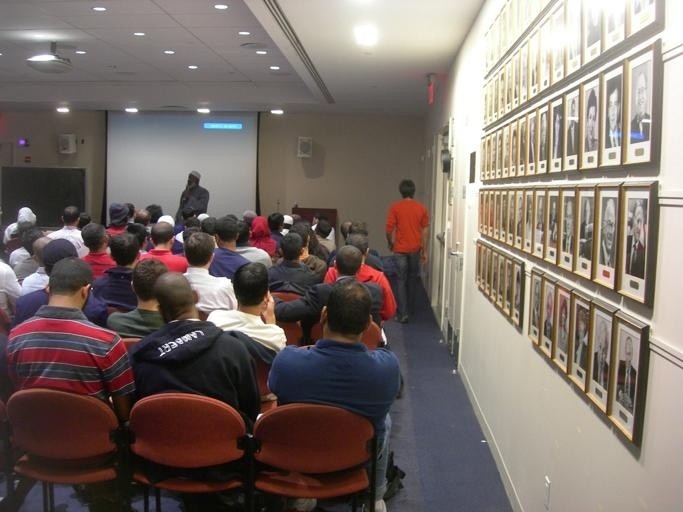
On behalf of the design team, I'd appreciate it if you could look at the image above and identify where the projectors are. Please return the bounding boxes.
[28,56,72,74]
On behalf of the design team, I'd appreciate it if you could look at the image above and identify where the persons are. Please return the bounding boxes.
[476,0,656,415]
[384,179,430,323]
[1,170,400,512]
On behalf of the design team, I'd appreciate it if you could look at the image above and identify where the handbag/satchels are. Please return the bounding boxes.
[350,451,404,506]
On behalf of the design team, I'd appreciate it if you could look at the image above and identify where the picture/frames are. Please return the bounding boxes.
[476,0,667,459]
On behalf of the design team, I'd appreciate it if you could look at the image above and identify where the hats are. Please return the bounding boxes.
[284,214,293,225]
[109,202,129,223]
[42,239,78,266]
[191,171,200,179]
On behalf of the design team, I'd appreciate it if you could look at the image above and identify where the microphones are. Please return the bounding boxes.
[186,180,192,192]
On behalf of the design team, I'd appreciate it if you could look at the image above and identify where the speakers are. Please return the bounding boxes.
[296,136,313,158]
[56,133,77,156]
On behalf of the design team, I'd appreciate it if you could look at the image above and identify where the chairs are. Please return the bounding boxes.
[125,393,248,511]
[249,402,376,512]
[256,288,382,409]
[5,389,122,512]
